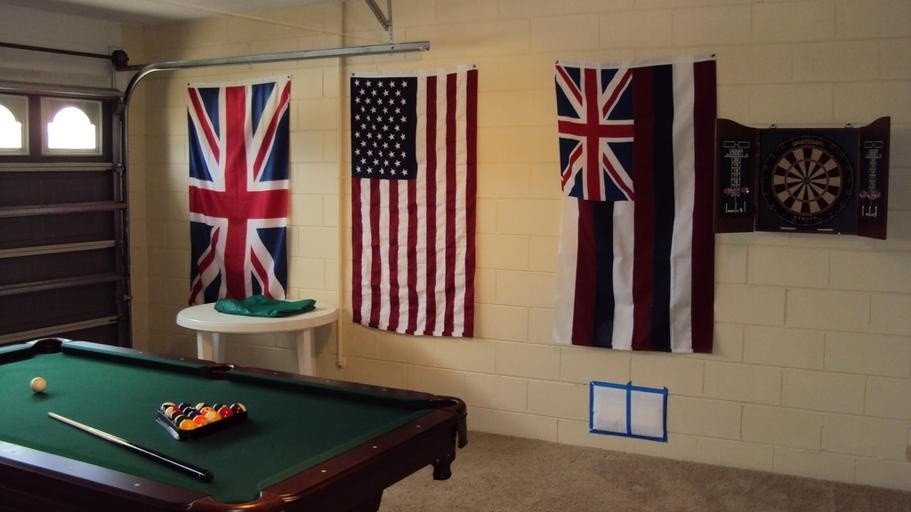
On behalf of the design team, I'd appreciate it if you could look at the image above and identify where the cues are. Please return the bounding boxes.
[49,411,213,480]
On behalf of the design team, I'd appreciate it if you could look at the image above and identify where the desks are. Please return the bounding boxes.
[0,337,469,511]
[176,301,338,378]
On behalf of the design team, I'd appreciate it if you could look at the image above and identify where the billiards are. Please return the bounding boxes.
[30,377,47,393]
[161,401,246,430]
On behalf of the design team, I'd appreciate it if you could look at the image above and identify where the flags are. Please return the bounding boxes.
[186,73,291,308]
[554,52,718,356]
[350,62,481,340]
